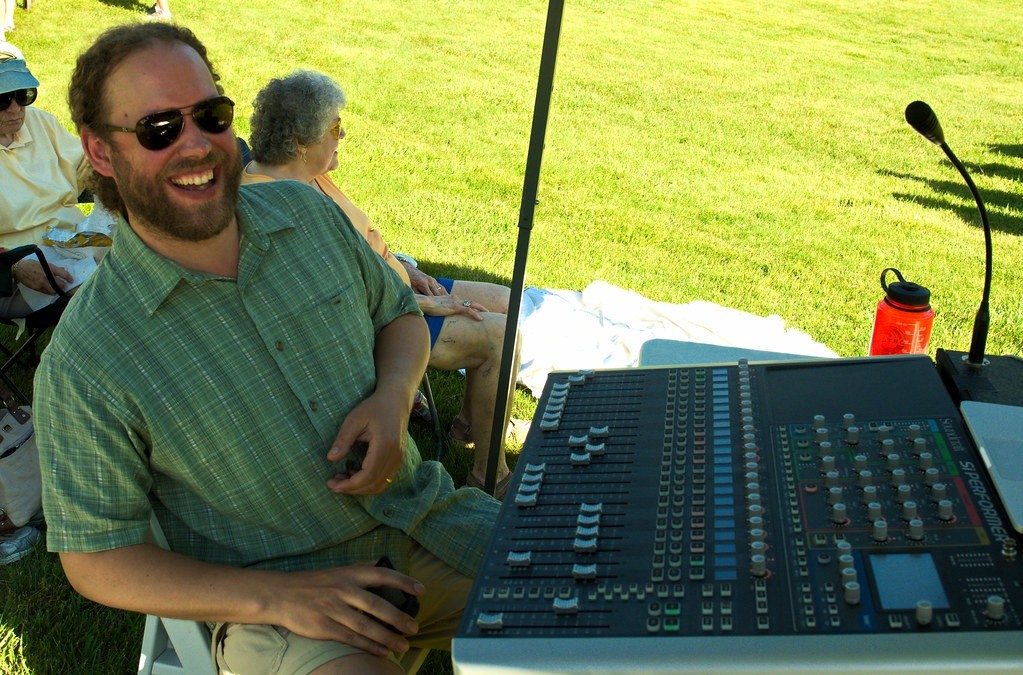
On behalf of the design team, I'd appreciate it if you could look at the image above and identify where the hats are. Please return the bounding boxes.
[0,59,39,94]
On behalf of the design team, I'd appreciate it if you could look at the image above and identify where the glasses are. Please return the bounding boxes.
[0,88,37,111]
[101,97,234,150]
[326,118,341,140]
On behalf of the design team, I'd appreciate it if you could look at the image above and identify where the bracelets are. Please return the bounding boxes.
[12,261,18,272]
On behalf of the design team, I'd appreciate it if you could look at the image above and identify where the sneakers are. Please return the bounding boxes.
[0,527,44,565]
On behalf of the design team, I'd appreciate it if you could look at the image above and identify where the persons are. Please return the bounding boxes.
[239,70,522,502]
[32,21,502,675]
[0,41,100,318]
[144,0,172,20]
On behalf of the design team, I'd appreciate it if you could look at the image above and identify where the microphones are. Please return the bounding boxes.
[905,101,992,364]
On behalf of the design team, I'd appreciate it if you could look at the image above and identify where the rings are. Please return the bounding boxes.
[463,301,471,306]
[438,287,441,290]
[386,478,392,484]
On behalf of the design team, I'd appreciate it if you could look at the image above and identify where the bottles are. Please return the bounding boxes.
[870,267,934,356]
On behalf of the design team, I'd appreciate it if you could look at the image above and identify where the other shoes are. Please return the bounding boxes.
[144,10,171,20]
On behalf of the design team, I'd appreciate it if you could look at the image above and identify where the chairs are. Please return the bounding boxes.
[137,506,435,674]
[0,244,70,367]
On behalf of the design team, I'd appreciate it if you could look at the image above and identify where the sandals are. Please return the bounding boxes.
[466,471,513,501]
[448,414,525,449]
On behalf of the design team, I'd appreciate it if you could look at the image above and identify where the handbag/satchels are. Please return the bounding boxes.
[0,379,43,527]
